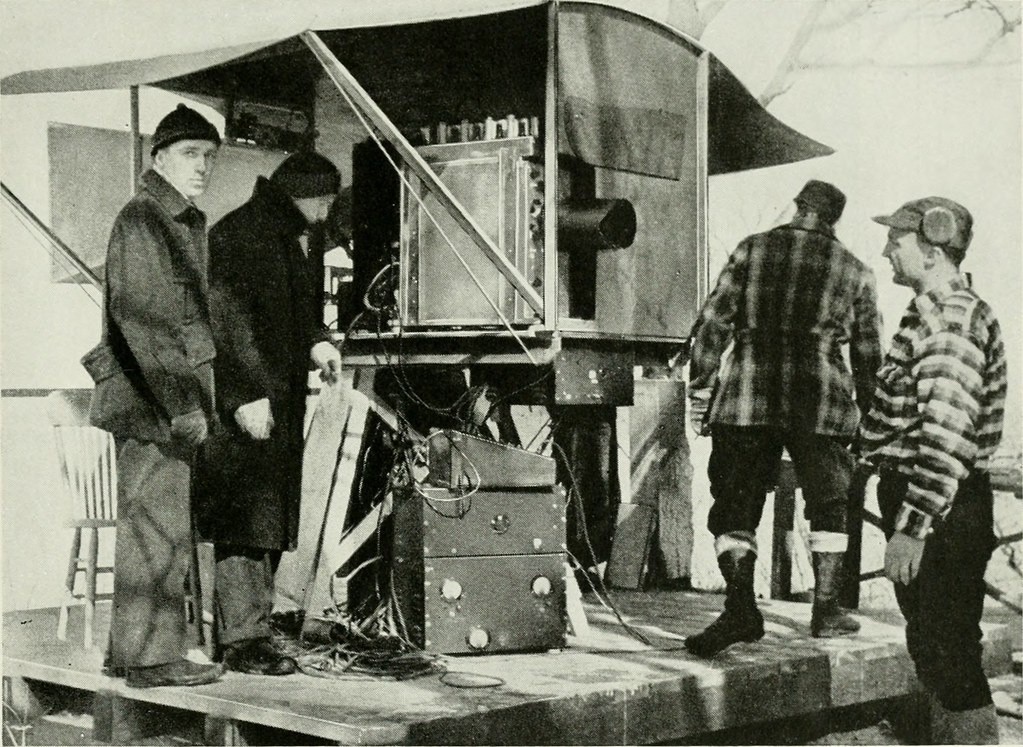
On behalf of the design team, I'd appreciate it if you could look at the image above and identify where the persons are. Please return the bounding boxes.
[81,102,222,688]
[205,150,344,674]
[851,196,1010,746]
[683,177,881,656]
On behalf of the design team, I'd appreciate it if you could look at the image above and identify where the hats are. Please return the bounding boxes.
[872,196,973,252]
[793,180,848,222]
[150,102,222,156]
[269,151,341,200]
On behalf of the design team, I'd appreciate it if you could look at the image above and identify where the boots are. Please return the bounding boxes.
[812,548,862,641]
[684,550,766,658]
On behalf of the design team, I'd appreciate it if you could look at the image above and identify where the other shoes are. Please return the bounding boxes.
[104,658,130,677]
[126,657,228,689]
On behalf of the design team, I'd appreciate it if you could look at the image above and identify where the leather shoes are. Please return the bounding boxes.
[214,640,296,676]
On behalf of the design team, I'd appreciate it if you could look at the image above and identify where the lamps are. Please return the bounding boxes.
[278,128,304,156]
[230,110,260,147]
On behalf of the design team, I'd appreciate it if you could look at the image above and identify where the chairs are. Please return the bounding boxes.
[46,386,206,649]
